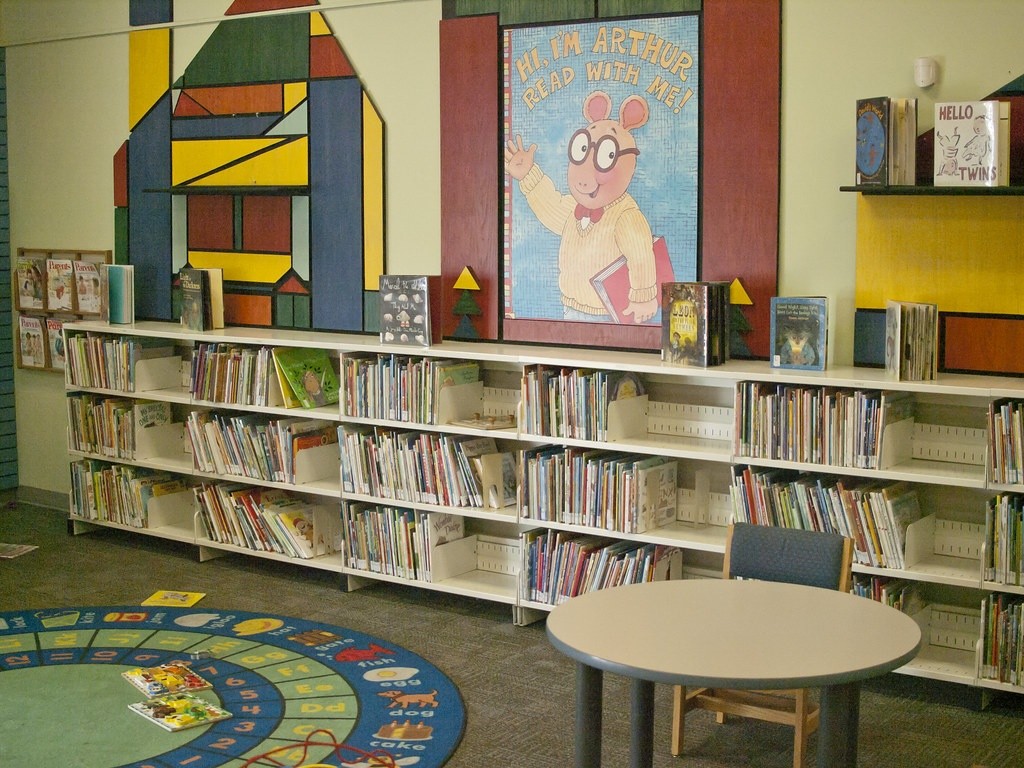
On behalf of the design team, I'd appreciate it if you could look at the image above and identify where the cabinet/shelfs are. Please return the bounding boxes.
[60,318,1024,711]
[14,246,113,373]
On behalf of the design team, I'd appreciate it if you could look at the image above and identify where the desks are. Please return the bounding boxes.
[544,580,922,768]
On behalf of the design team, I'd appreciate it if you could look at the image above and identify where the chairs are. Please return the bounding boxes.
[672,523,854,768]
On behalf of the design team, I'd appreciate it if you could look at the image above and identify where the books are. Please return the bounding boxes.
[0,543,39,559]
[934,100,1011,187]
[855,96,918,187]
[127,693,234,733]
[16,257,1024,689]
[121,663,214,699]
